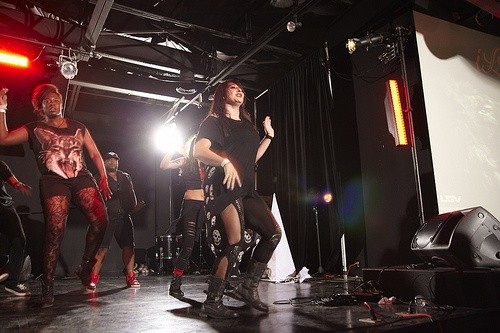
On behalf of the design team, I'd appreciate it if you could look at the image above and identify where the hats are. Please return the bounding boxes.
[103,151,120,161]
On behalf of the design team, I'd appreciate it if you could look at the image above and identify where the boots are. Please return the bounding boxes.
[76,255,97,290]
[199,276,240,318]
[41,278,55,308]
[169,273,184,295]
[233,258,268,313]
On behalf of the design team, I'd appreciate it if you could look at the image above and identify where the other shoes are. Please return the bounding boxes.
[126,272,140,287]
[90,273,99,288]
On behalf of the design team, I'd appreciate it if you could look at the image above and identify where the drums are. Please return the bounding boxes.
[154,234,182,260]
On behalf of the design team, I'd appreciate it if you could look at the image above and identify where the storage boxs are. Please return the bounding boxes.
[154,235,183,259]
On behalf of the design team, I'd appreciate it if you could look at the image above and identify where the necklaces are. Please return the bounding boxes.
[58,121,64,128]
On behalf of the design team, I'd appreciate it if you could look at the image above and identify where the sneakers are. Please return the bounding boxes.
[5,281,31,297]
[0,272,8,282]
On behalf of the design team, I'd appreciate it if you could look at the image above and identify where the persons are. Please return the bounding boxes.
[194,79,282,318]
[160,135,235,297]
[0,160,32,296]
[0,82,112,309]
[88,152,141,290]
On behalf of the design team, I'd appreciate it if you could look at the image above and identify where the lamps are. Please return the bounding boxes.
[56,47,78,79]
[176,64,196,94]
[346,31,388,53]
[388,78,412,146]
[286,14,302,32]
[208,86,216,101]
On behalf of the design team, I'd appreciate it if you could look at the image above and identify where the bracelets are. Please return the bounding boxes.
[267,135,273,140]
[221,159,230,167]
[0,105,7,112]
